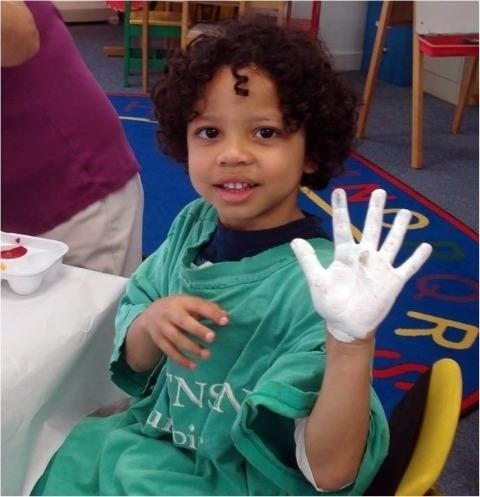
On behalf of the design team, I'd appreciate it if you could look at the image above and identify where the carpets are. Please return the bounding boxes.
[108,92,479,420]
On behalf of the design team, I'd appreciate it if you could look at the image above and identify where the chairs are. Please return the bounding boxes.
[362,359,464,495]
[285,1,319,40]
[121,6,191,86]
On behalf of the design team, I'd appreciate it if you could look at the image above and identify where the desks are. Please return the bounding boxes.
[1,264,131,496]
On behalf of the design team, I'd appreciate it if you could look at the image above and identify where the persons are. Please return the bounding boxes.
[0,0,144,280]
[29,8,432,495]
